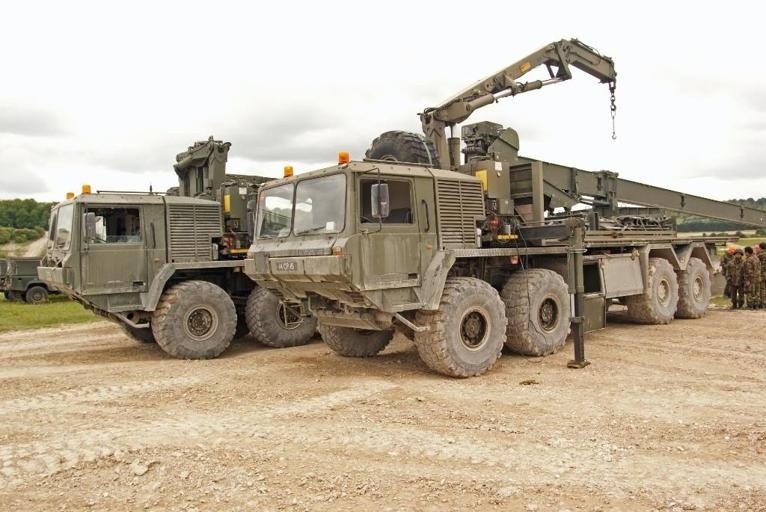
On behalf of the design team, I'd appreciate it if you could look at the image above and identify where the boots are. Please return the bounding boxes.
[731,304,766,311]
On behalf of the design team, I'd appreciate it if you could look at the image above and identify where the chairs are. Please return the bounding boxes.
[382,207,411,223]
[117,214,137,242]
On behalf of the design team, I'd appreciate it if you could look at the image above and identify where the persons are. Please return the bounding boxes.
[719,241,766,312]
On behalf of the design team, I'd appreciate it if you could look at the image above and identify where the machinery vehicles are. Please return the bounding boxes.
[35,134,322,361]
[0,204,80,304]
[242,33,766,381]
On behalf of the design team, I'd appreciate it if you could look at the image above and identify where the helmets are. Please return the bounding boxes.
[732,248,744,256]
